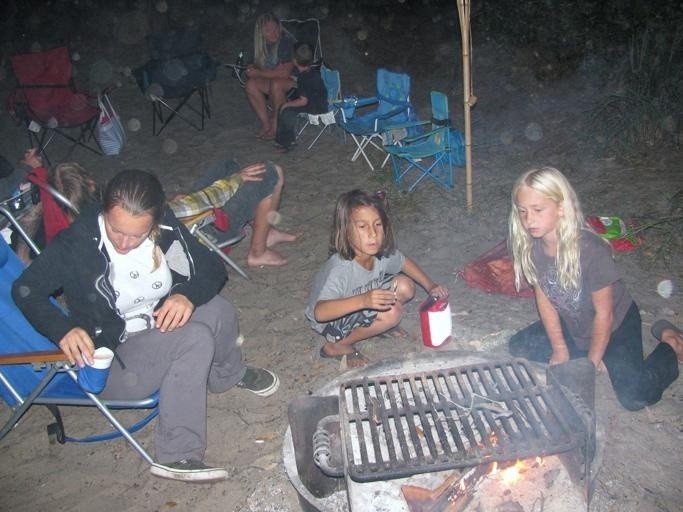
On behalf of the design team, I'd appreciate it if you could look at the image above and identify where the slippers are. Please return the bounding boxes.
[319,345,368,367]
[382,328,406,339]
[650,320,683,342]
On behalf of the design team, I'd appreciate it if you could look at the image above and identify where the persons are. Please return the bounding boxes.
[303,189,451,368]
[507,165,682,411]
[42,157,302,267]
[9,167,281,483]
[266,40,329,155]
[242,14,300,141]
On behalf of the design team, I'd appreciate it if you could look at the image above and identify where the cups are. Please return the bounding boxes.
[82,347,114,369]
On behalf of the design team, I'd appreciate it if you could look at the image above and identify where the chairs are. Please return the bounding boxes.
[9,18,221,167]
[280,65,455,193]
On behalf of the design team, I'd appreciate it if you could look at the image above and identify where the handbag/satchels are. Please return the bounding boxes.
[95,111,126,156]
[408,104,425,144]
[434,127,465,167]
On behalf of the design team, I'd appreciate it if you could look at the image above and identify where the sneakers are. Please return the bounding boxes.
[150,460,227,484]
[239,366,279,396]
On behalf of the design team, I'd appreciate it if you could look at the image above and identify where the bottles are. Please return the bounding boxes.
[237,48,249,65]
